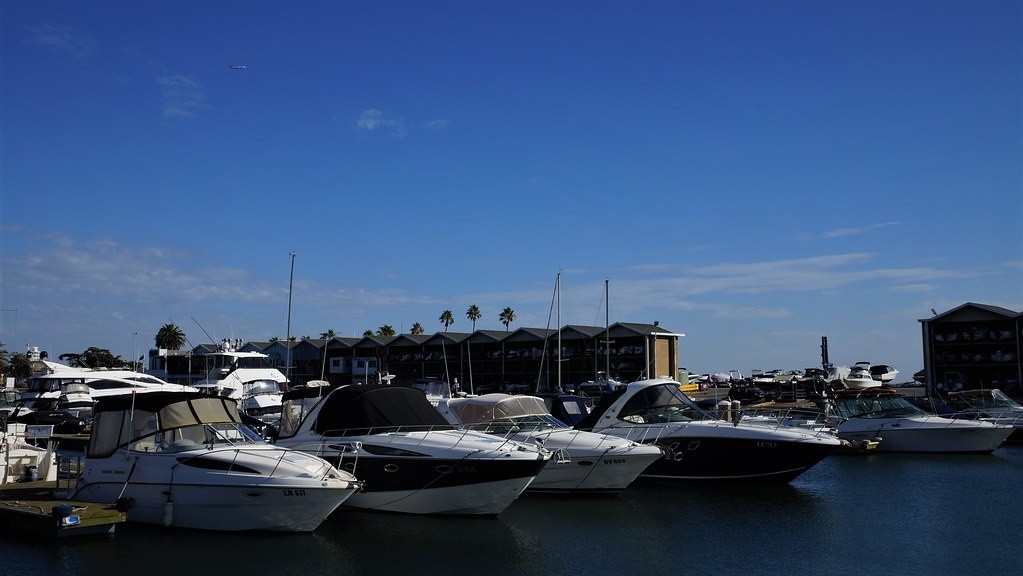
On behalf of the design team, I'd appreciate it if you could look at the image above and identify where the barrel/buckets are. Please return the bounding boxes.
[25,469,37,482]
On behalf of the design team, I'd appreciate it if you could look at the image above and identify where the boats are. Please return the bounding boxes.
[237,379,298,421]
[910,389,1023,432]
[843,361,882,390]
[549,379,850,494]
[752,370,785,390]
[411,379,451,407]
[476,381,532,393]
[66,388,367,531]
[239,384,555,518]
[870,365,899,384]
[0,360,201,483]
[912,369,925,383]
[749,388,1023,457]
[192,338,290,401]
[436,392,691,497]
[678,367,750,385]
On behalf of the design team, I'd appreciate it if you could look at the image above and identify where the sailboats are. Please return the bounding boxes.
[530,273,621,400]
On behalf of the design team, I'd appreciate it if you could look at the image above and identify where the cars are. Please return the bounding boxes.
[728,385,764,400]
